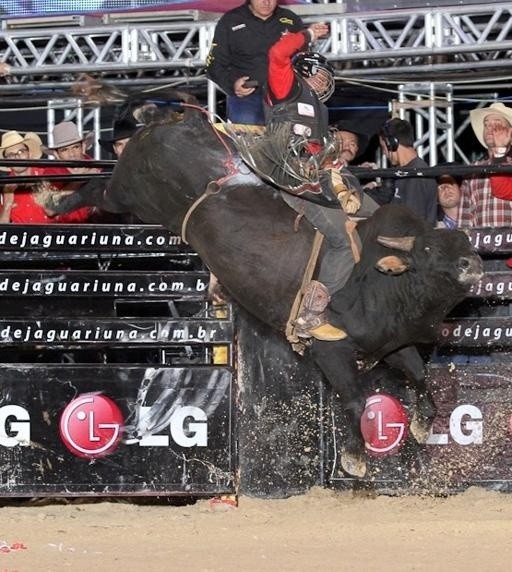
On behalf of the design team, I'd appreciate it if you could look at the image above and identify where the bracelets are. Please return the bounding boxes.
[307,27,314,42]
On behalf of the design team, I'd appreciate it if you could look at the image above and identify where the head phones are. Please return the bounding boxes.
[383,117,401,152]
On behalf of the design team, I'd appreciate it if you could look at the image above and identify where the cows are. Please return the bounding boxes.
[30,74,486,478]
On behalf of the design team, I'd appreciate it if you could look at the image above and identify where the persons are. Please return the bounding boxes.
[269,23,363,343]
[2,120,213,362]
[333,104,512,360]
[207,0,310,124]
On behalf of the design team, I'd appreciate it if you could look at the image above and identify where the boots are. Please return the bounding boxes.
[297,278,348,343]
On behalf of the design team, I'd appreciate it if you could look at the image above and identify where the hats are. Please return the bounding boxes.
[468,103,512,150]
[40,120,95,158]
[97,118,139,154]
[0,131,41,174]
[334,119,369,140]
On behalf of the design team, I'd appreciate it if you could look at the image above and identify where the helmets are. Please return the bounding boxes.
[291,51,338,103]
